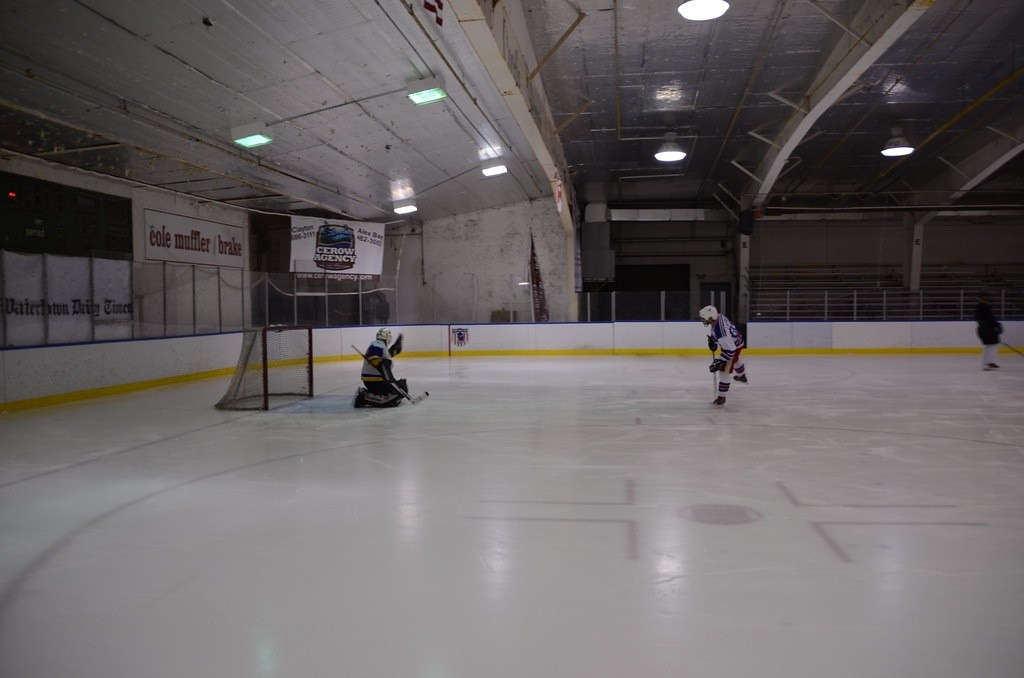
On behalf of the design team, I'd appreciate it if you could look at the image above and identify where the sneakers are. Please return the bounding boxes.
[734,373,748,384]
[713,396,726,409]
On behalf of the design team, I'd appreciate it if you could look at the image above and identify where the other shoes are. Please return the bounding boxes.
[983,363,1001,371]
[351,388,366,408]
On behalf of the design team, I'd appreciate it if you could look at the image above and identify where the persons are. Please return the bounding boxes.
[351,328,408,407]
[699,305,748,409]
[977,307,1003,371]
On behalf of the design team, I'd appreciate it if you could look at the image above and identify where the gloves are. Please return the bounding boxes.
[709,359,723,372]
[709,340,717,352]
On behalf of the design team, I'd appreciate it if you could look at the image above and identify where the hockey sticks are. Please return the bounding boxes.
[350,344,430,405]
[712,351,718,399]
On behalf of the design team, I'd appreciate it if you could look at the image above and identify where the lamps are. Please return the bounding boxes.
[393,200,417,215]
[406,76,448,107]
[480,159,508,176]
[654,142,688,162]
[880,138,915,157]
[231,122,274,150]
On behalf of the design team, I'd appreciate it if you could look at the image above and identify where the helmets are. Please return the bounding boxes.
[376,328,392,345]
[699,305,718,327]
